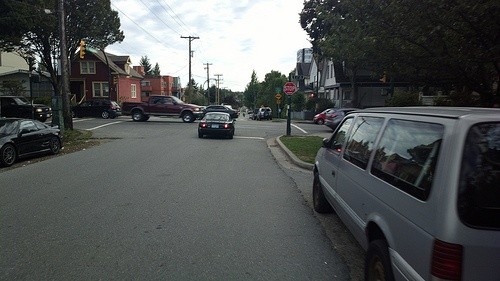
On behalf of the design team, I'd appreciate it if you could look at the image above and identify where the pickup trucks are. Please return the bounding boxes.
[122,95,203,123]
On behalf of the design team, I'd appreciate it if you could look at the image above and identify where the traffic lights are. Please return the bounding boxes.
[79,41,86,58]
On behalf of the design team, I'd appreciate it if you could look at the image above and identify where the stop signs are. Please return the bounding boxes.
[283,82,298,96]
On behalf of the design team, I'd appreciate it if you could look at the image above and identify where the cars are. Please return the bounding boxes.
[198,112,234,139]
[0,118,62,166]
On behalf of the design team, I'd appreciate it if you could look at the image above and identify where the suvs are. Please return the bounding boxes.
[71,99,120,119]
[249,107,273,121]
[314,109,331,125]
[324,109,360,130]
[205,105,239,122]
[0,96,52,122]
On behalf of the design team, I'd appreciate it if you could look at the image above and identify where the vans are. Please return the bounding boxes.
[312,107,500,281]
